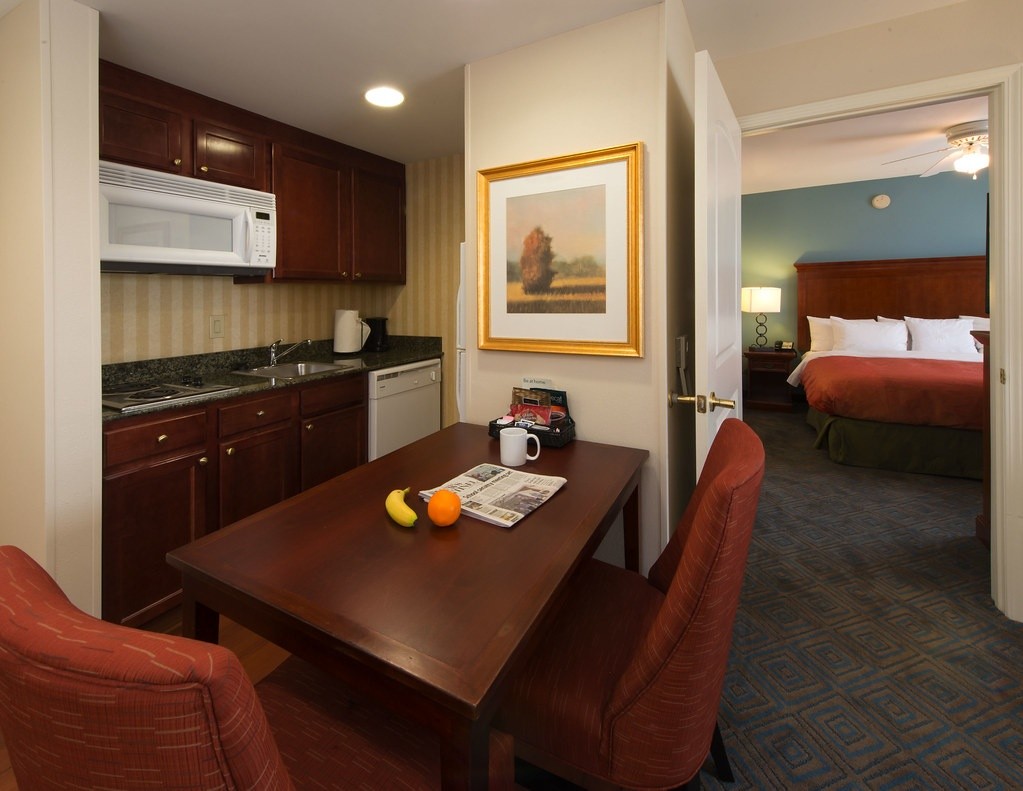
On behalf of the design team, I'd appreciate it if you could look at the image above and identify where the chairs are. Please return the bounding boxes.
[0,542,516,791]
[490,418,765,791]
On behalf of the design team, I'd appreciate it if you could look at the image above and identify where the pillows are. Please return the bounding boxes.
[805,314,990,354]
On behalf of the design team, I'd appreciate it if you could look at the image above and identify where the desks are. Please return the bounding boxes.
[166,423,649,791]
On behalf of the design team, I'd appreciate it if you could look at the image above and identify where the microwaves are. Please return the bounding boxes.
[100,160,276,276]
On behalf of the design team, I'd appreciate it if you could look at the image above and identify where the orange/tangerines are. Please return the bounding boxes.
[427,490,461,526]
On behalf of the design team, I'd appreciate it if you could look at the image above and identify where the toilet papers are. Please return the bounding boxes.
[333,309,371,353]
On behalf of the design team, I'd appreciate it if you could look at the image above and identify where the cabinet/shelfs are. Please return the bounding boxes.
[102,372,371,630]
[97,57,407,287]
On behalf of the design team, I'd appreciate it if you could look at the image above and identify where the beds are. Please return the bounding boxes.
[796,257,990,481]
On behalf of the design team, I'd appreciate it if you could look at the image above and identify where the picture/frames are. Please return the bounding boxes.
[477,144,646,360]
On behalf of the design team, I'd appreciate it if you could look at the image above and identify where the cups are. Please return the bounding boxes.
[550,411,566,430]
[500,427,541,467]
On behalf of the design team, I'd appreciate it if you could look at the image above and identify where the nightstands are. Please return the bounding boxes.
[743,352,802,412]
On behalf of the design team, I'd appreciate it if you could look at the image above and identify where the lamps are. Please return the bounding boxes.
[952,147,990,180]
[740,287,781,353]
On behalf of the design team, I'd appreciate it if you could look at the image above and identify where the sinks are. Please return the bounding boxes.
[231,364,354,379]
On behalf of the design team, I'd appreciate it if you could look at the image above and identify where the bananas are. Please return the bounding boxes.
[384,487,418,527]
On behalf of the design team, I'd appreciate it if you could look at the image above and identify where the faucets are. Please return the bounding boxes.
[269,337,310,366]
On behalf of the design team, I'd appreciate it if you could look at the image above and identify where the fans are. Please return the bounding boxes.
[881,119,990,180]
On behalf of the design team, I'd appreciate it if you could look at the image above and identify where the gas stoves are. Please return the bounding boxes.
[102,373,276,413]
